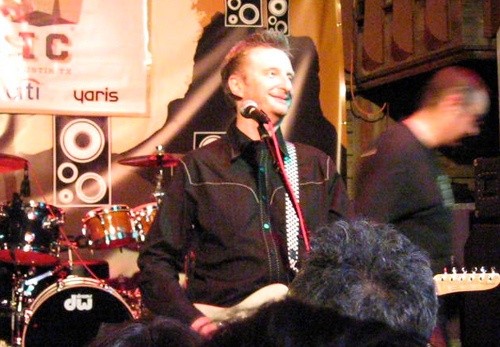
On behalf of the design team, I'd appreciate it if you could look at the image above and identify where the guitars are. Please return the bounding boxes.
[192,267,500,328]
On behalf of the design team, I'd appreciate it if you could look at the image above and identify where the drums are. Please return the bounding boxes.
[15,275,137,347]
[82,204,135,250]
[0,200,65,266]
[132,202,159,249]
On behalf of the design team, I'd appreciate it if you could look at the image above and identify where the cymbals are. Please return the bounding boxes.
[118,154,187,168]
[0,153,29,175]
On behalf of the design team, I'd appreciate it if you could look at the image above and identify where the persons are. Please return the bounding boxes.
[266,218,439,347]
[84,319,209,347]
[346,63,489,341]
[135,30,356,338]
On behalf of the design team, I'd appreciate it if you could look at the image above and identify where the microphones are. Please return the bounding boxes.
[19,163,31,198]
[240,100,271,123]
